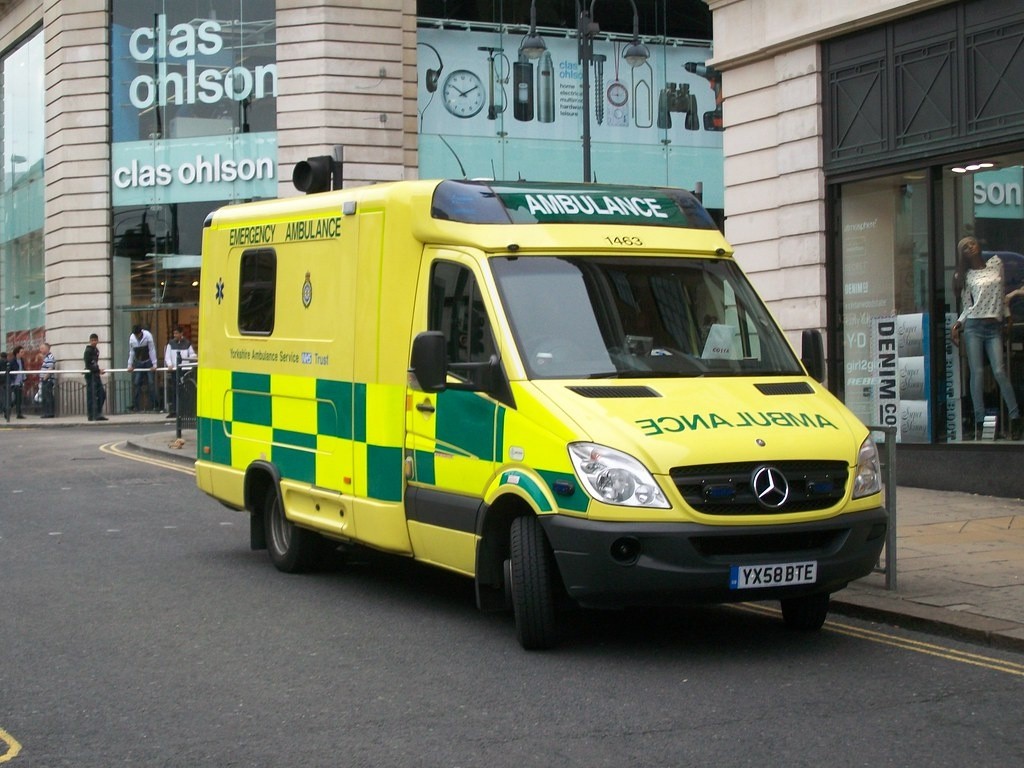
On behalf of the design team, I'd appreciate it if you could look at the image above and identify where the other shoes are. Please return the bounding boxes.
[16,415,25,418]
[41,415,54,418]
[95,416,109,421]
[127,405,139,411]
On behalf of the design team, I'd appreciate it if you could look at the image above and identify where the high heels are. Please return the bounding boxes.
[1011,419,1021,440]
[976,422,983,441]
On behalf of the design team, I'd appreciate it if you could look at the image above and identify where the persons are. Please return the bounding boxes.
[38,343,56,418]
[4,345,27,420]
[0,352,9,414]
[949,234,1023,441]
[83,333,109,421]
[126,324,159,412]
[165,327,197,418]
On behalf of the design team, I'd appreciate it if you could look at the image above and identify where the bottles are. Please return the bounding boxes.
[536,50,557,124]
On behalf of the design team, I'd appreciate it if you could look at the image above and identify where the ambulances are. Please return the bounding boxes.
[194,178,889,651]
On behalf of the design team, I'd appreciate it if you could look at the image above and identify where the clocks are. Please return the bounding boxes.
[441,70,486,119]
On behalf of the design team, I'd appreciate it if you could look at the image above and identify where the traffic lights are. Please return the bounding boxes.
[291,154,334,193]
